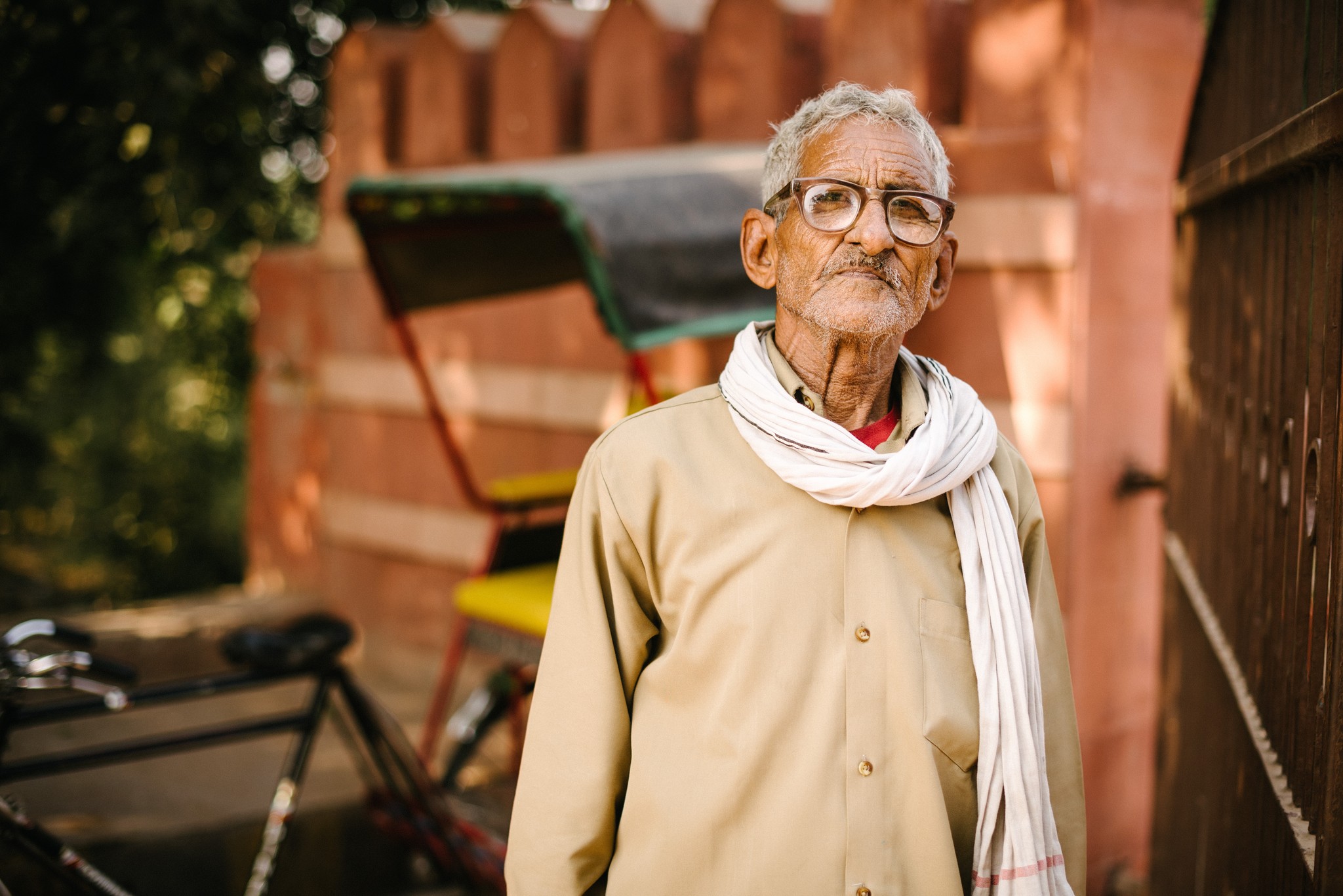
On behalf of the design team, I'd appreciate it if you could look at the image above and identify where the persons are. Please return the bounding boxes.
[504,80,1089,896]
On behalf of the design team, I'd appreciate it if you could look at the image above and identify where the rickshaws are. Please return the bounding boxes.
[0,144,775,896]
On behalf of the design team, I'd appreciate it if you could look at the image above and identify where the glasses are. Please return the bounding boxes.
[762,177,956,248]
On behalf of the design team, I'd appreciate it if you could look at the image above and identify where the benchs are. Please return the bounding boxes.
[452,465,577,642]
